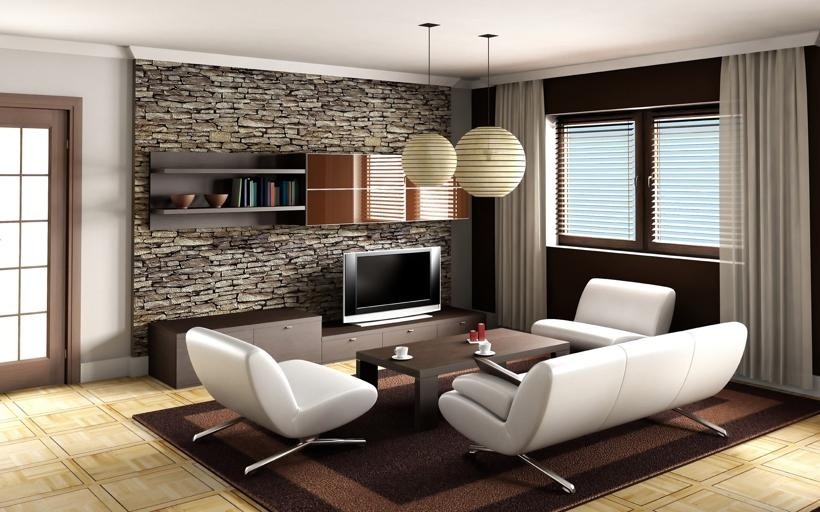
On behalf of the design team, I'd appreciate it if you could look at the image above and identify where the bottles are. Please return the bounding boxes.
[470,329,476,339]
[477,322,486,340]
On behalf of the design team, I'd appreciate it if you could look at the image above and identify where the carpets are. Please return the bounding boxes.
[133,362,820,512]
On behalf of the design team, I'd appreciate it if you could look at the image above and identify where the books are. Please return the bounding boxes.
[212,175,301,207]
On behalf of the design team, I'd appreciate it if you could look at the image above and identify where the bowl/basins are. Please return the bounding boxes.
[204,193,227,209]
[170,192,195,209]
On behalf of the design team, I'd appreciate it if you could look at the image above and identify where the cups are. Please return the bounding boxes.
[478,341,492,353]
[394,345,409,358]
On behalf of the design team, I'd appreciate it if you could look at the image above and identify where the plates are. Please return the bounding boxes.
[391,353,414,360]
[466,337,488,344]
[473,350,497,357]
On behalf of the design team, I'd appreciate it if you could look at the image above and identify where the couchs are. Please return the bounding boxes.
[530,277,677,353]
[183,326,380,474]
[446,321,749,495]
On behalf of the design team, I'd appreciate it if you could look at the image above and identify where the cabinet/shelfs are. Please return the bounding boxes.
[277,154,468,225]
[147,147,304,226]
[318,304,487,364]
[147,309,322,388]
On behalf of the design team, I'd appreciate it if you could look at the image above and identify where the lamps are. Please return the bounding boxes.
[398,18,458,188]
[454,33,528,199]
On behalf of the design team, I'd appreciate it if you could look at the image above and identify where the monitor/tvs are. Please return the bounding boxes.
[343,246,442,328]
